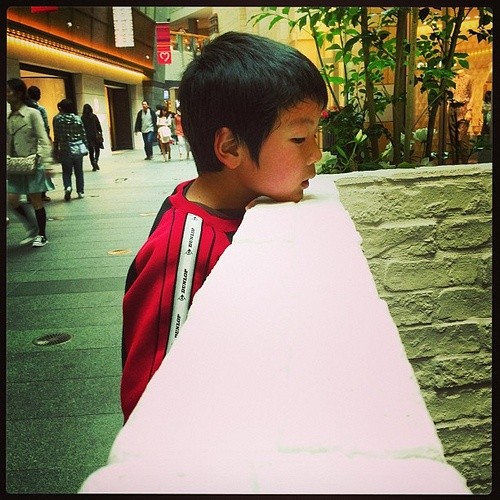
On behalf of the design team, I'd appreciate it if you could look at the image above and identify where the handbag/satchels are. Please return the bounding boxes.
[66,140,88,158]
[7,154,41,175]
[158,125,171,143]
[97,133,104,149]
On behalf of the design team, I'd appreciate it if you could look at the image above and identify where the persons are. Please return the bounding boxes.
[154,105,173,162]
[174,107,190,160]
[53,99,88,201]
[134,100,157,161]
[7,78,55,247]
[120,32,328,427]
[26,86,53,200]
[81,104,104,171]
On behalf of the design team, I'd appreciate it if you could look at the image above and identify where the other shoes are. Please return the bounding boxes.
[161,154,168,162]
[91,160,101,171]
[19,231,33,247]
[31,235,45,247]
[43,194,51,201]
[144,154,153,160]
[64,186,73,202]
[179,153,183,160]
[78,193,84,197]
